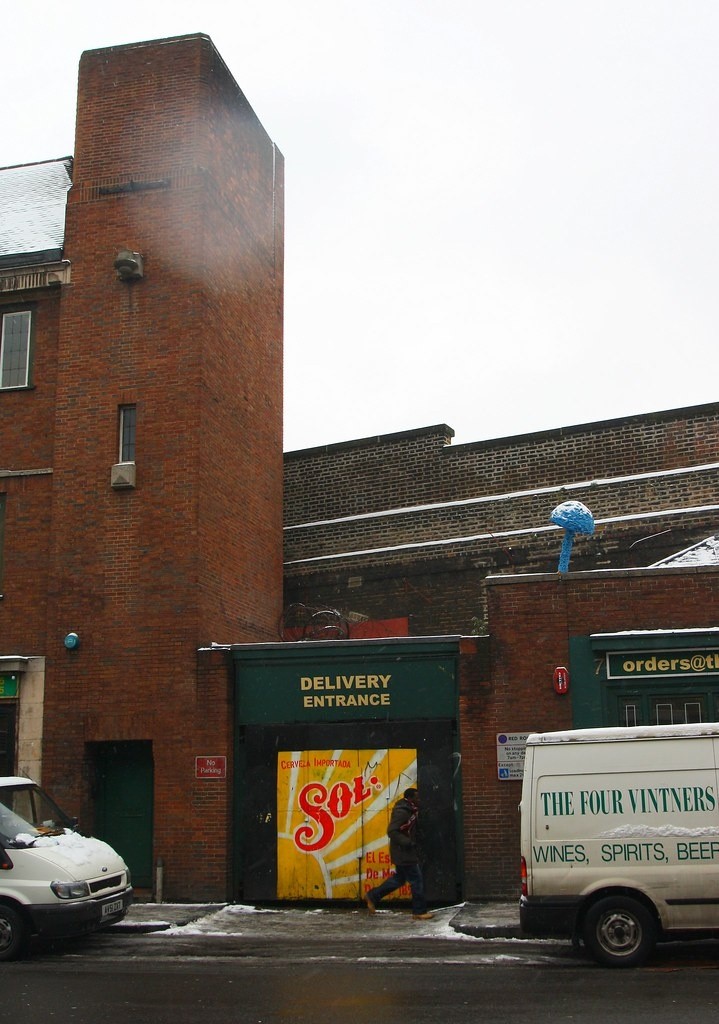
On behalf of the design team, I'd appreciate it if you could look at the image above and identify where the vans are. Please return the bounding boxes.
[0,775,133,960]
[519,726,718,959]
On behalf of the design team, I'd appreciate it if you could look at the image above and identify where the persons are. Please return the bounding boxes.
[364,788,435,919]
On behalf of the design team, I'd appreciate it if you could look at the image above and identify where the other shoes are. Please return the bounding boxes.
[365,894,375,914]
[412,912,434,920]
